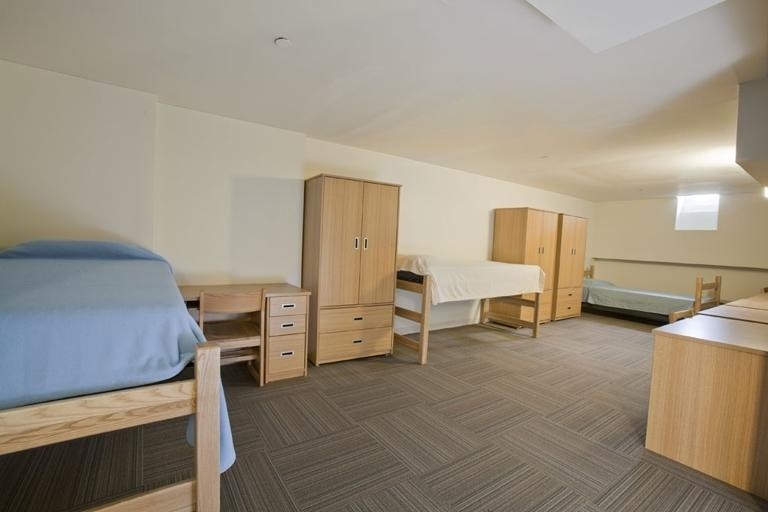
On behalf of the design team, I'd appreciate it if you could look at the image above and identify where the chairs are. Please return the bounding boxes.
[696,276,721,313]
[196,288,266,387]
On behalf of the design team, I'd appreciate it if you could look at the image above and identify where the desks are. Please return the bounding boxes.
[646,292,768,501]
[177,283,312,383]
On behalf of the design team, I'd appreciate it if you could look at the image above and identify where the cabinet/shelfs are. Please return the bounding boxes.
[492,209,559,329]
[553,207,590,323]
[303,174,402,368]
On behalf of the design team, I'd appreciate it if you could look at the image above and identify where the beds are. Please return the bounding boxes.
[0,239,237,512]
[395,255,545,364]
[583,265,711,322]
